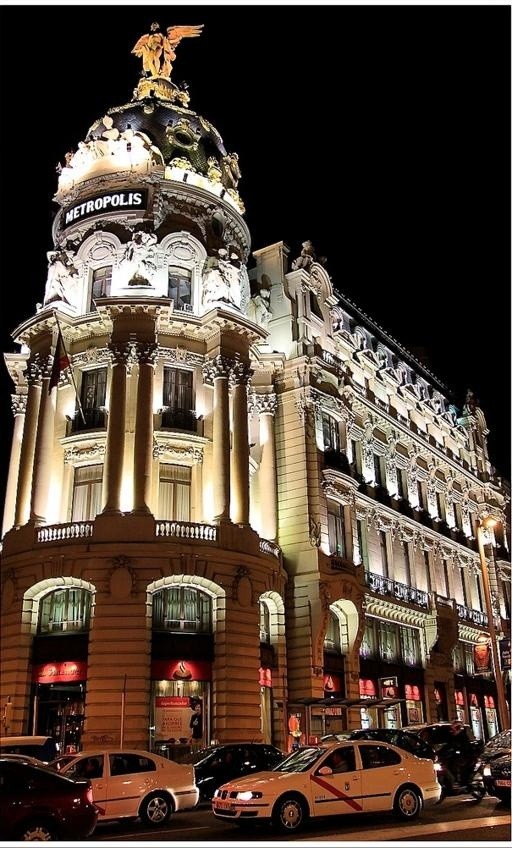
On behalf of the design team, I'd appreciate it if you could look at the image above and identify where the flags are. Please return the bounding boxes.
[46,332,70,396]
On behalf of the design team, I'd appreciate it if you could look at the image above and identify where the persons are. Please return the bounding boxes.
[140,21,176,77]
[189,702,202,742]
[439,721,475,794]
[332,753,348,774]
[84,759,101,778]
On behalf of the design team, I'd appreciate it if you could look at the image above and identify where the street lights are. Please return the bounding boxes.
[475,513,509,730]
